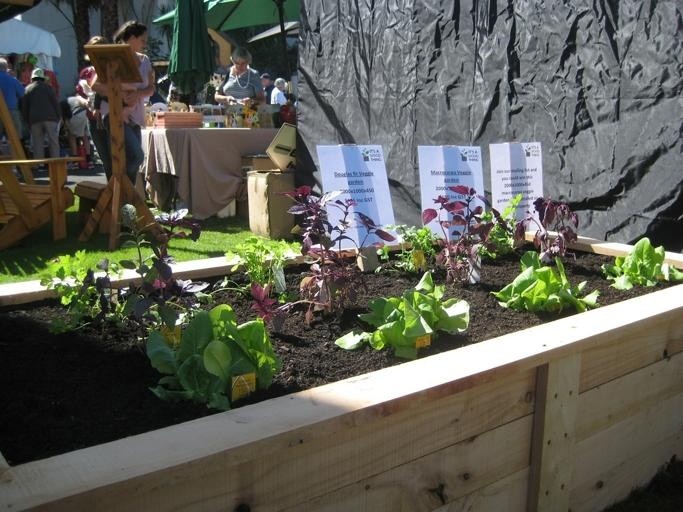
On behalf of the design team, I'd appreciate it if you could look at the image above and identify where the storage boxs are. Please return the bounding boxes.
[235,119,306,238]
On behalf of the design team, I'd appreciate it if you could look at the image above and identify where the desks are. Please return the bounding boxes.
[141,125,292,217]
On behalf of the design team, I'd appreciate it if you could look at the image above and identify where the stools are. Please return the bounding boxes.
[74,181,108,218]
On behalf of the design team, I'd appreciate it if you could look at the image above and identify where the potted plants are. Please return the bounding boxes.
[0,180,683,511]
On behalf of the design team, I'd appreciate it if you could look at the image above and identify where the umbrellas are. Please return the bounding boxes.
[167,0,214,103]
[152,0,300,81]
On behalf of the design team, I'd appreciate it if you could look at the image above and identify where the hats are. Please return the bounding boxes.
[29,68,50,82]
